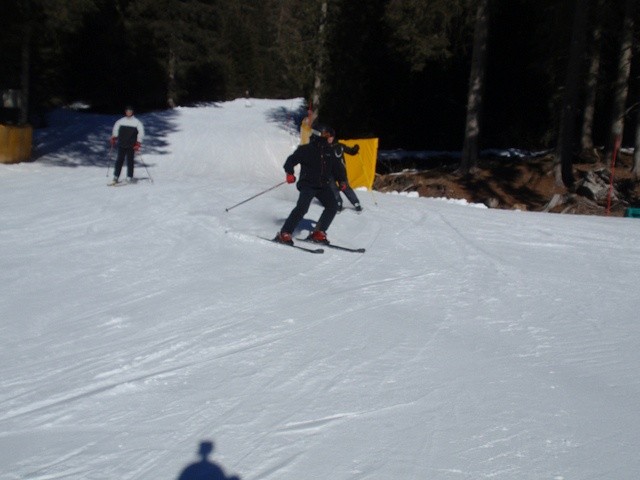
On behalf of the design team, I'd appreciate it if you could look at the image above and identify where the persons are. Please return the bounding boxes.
[327,141,362,212]
[277,126,346,247]
[111,106,143,184]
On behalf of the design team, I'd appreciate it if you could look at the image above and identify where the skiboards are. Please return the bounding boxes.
[257,235,366,254]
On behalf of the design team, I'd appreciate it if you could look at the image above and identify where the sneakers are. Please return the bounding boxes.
[112,179,118,184]
[337,201,342,211]
[311,230,330,245]
[123,179,128,185]
[277,230,294,245]
[354,202,360,211]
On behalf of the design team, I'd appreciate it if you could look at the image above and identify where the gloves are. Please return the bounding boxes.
[111,137,115,144]
[133,143,140,151]
[287,172,295,183]
[340,184,347,191]
